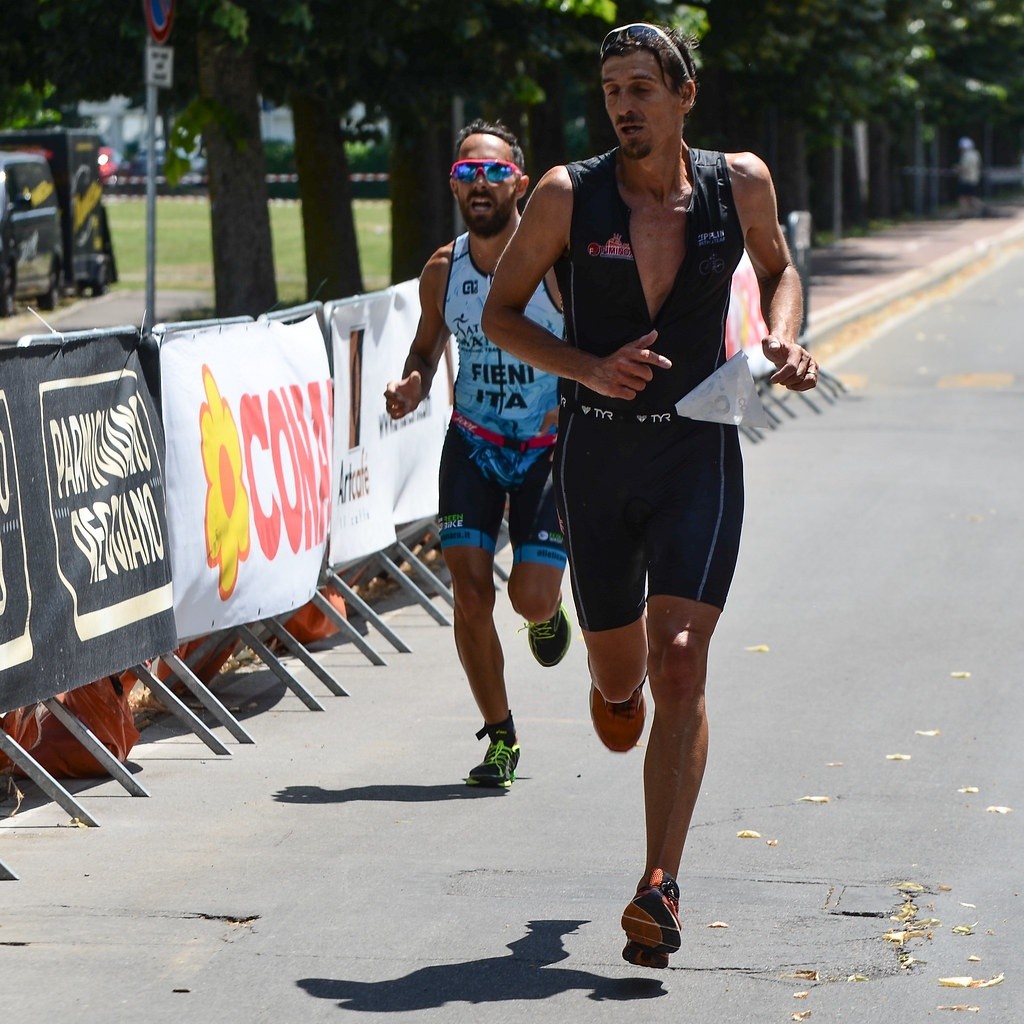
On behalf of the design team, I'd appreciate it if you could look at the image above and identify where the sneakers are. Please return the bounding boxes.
[589,683,647,753]
[518,603,571,667]
[622,867,681,968]
[466,728,520,787]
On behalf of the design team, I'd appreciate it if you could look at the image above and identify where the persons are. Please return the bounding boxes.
[955,137,992,219]
[481,24,819,967]
[381,120,564,785]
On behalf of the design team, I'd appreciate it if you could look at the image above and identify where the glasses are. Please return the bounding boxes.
[450,159,522,182]
[602,23,691,81]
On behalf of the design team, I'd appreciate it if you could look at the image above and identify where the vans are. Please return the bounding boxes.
[0,123,117,319]
